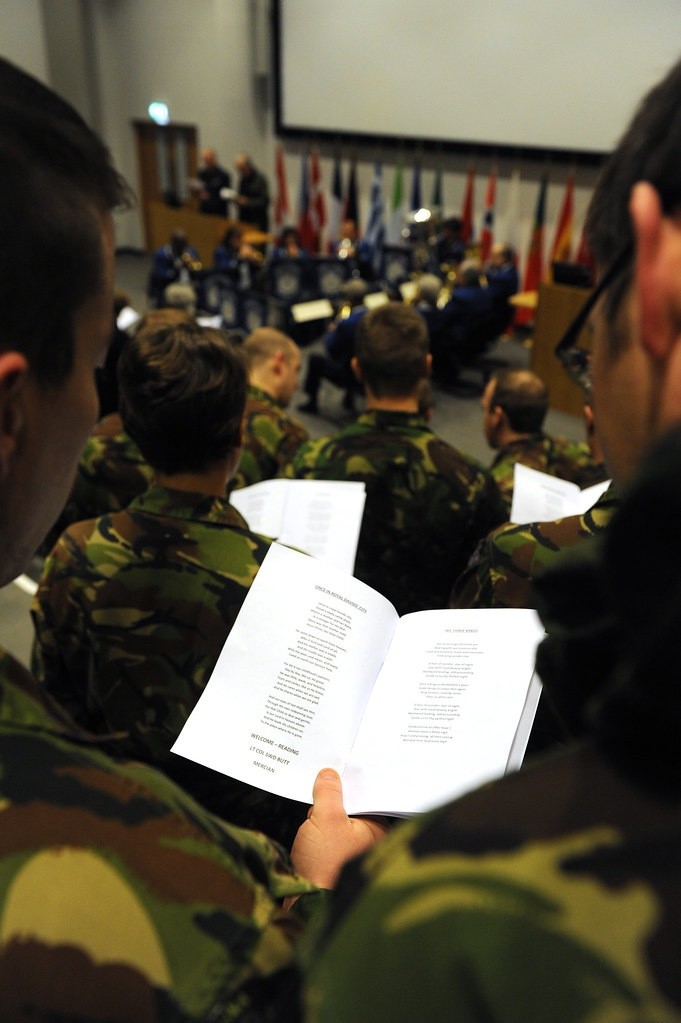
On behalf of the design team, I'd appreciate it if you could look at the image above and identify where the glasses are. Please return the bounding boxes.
[553,242,634,395]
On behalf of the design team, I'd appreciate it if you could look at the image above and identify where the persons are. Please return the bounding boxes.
[145,149,519,415]
[450,472,621,607]
[307,57,681,1023]
[1,57,384,1023]
[481,368,591,485]
[234,327,310,487]
[36,307,202,554]
[29,320,307,839]
[498,381,607,511]
[273,303,507,613]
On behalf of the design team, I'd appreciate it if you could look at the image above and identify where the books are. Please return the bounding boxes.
[229,478,367,575]
[509,462,612,524]
[171,539,544,821]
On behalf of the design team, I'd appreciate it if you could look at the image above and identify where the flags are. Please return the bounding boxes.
[274,139,592,343]
